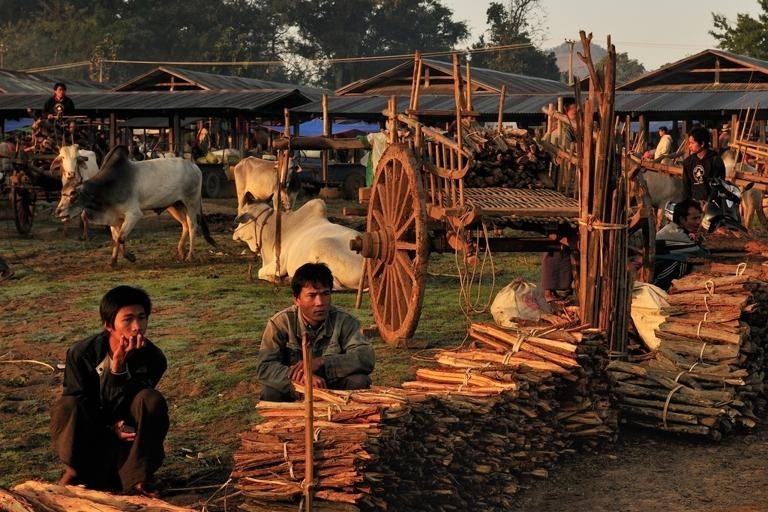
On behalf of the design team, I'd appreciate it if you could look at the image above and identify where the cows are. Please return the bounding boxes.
[641,157,684,233]
[232,198,383,292]
[53,144,218,271]
[720,150,761,229]
[50,143,101,241]
[234,155,303,217]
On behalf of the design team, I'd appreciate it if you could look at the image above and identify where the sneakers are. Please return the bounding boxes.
[55,468,162,500]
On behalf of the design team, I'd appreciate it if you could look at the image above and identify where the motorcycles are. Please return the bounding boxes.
[665,176,754,234]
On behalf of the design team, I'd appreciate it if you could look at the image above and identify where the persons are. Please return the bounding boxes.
[653,125,673,161]
[52,284,177,498]
[645,199,711,297]
[679,126,726,213]
[540,94,597,190]
[718,122,730,149]
[43,81,78,140]
[542,228,576,312]
[254,260,376,404]
